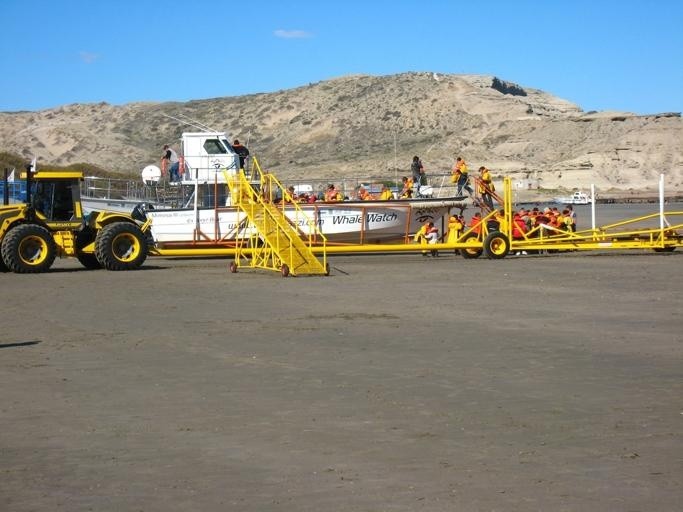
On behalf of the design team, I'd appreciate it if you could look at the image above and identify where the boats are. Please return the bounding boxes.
[553,191,597,205]
[79,175,203,220]
[146,112,469,244]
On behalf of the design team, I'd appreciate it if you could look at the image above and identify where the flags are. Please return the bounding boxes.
[5,167,15,183]
[28,154,36,172]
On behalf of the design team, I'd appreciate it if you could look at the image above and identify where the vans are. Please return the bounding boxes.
[294,184,314,196]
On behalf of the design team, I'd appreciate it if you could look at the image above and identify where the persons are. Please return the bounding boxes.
[451,157,495,211]
[376,177,412,200]
[411,155,427,185]
[231,140,249,168]
[420,204,576,259]
[276,180,375,203]
[159,144,180,183]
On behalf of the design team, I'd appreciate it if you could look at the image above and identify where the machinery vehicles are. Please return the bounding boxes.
[0,162,155,272]
